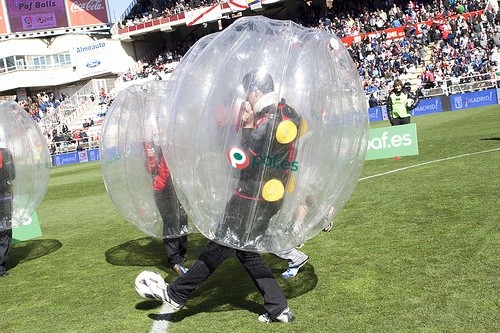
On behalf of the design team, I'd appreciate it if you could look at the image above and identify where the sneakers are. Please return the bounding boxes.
[144,276,184,311]
[258,306,295,323]
[324,206,335,232]
[173,264,189,276]
[157,254,181,270]
[280,251,309,278]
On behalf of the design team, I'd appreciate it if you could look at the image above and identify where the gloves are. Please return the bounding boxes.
[406,106,414,110]
[390,118,395,124]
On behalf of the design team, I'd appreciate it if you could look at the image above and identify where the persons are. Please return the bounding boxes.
[295,0,500,108]
[18,91,70,123]
[143,142,190,277]
[134,70,301,323]
[387,79,420,126]
[268,192,334,278]
[0,148,16,277]
[89,87,115,117]
[42,118,94,155]
[123,41,192,82]
[117,0,221,29]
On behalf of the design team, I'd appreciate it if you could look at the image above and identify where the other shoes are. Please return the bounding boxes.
[0,266,9,277]
